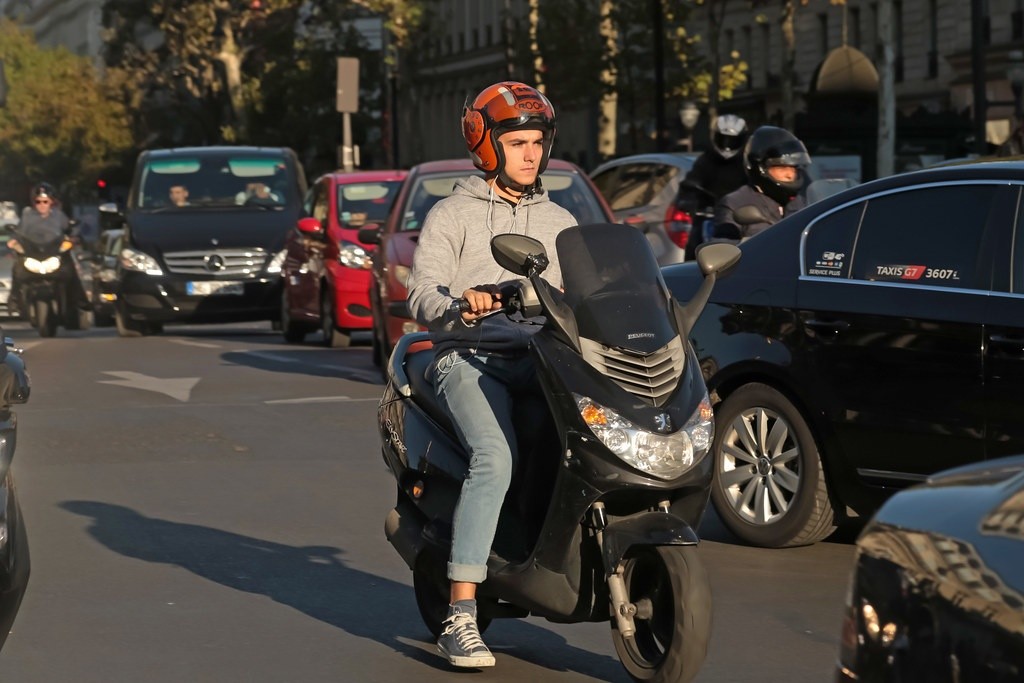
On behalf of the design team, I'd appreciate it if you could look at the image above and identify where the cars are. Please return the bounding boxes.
[278,168,410,349]
[828,453,1024,683]
[586,150,707,269]
[622,159,1024,551]
[0,221,127,338]
[357,155,650,390]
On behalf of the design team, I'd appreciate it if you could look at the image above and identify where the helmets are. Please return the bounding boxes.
[713,114,747,160]
[744,125,810,201]
[35,183,53,197]
[464,82,554,175]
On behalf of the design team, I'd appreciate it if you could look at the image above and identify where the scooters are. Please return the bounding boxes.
[374,220,744,683]
[6,219,78,338]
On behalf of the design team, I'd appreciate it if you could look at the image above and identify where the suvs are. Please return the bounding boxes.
[97,144,314,338]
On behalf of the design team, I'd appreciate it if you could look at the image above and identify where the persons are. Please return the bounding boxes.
[403,81,608,668]
[673,114,829,261]
[345,200,371,229]
[6,191,93,321]
[234,176,282,206]
[167,183,193,210]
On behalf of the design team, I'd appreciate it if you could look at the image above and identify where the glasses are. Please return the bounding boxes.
[36,200,50,204]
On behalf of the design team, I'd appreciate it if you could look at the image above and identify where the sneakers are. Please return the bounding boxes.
[437,610,496,667]
